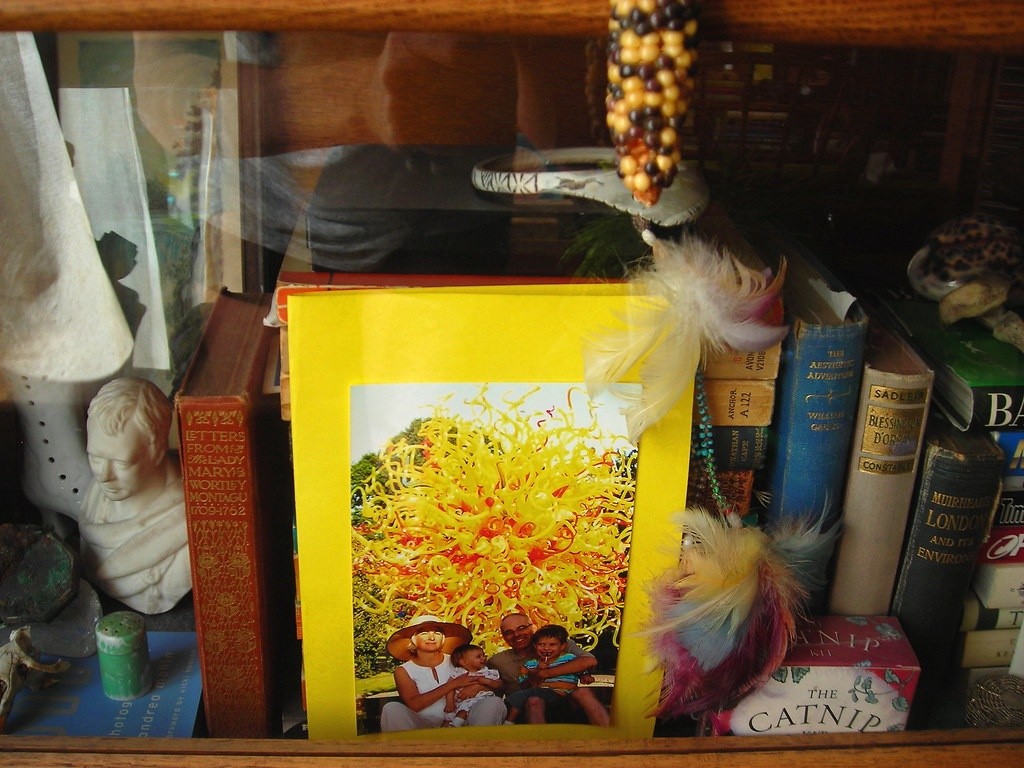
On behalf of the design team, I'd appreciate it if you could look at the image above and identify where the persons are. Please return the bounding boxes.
[79,376,193,615]
[503,625,596,725]
[443,644,503,727]
[381,615,503,732]
[486,613,610,728]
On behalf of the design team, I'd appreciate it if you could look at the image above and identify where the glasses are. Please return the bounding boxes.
[502,624,532,636]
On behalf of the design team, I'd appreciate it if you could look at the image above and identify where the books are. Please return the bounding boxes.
[176,286,278,739]
[269,193,1024,727]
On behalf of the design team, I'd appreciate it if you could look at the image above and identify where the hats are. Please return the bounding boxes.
[385,615,473,661]
